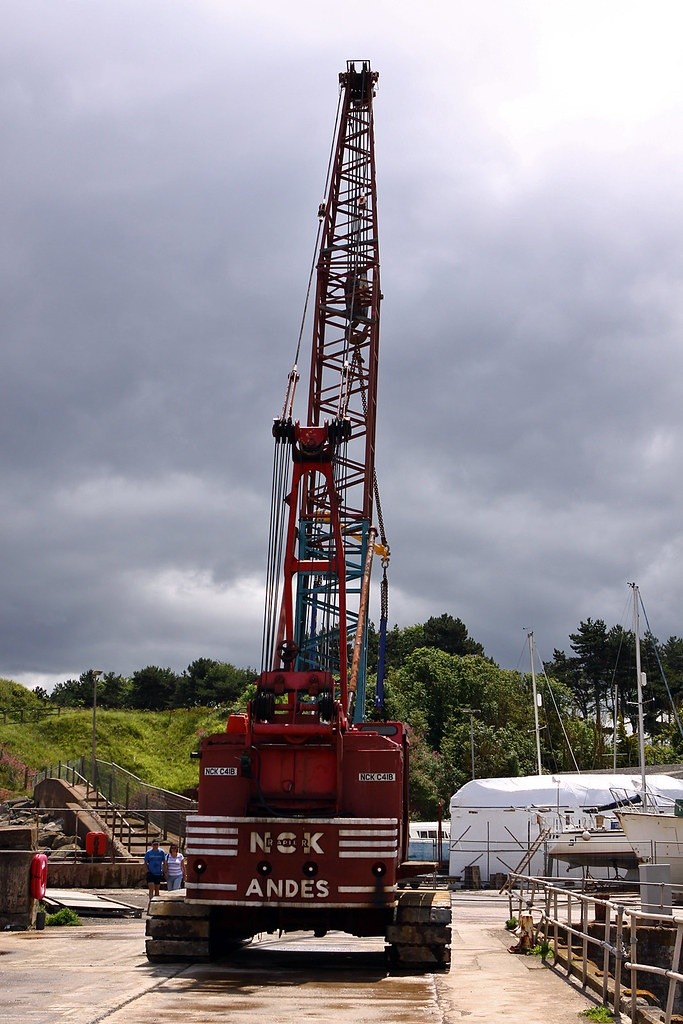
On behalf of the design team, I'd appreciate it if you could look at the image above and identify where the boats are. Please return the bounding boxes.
[609,582,683,905]
[548,788,641,870]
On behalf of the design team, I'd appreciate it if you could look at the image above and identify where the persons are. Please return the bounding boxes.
[163,845,186,890]
[144,840,167,899]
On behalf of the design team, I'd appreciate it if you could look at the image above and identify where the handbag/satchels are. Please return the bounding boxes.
[162,854,169,880]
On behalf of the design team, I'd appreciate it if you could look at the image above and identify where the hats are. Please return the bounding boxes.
[152,838,159,845]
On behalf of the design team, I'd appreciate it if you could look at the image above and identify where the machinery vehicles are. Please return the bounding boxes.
[139,61,456,971]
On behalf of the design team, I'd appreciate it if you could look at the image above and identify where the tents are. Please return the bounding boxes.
[448,775,683,885]
